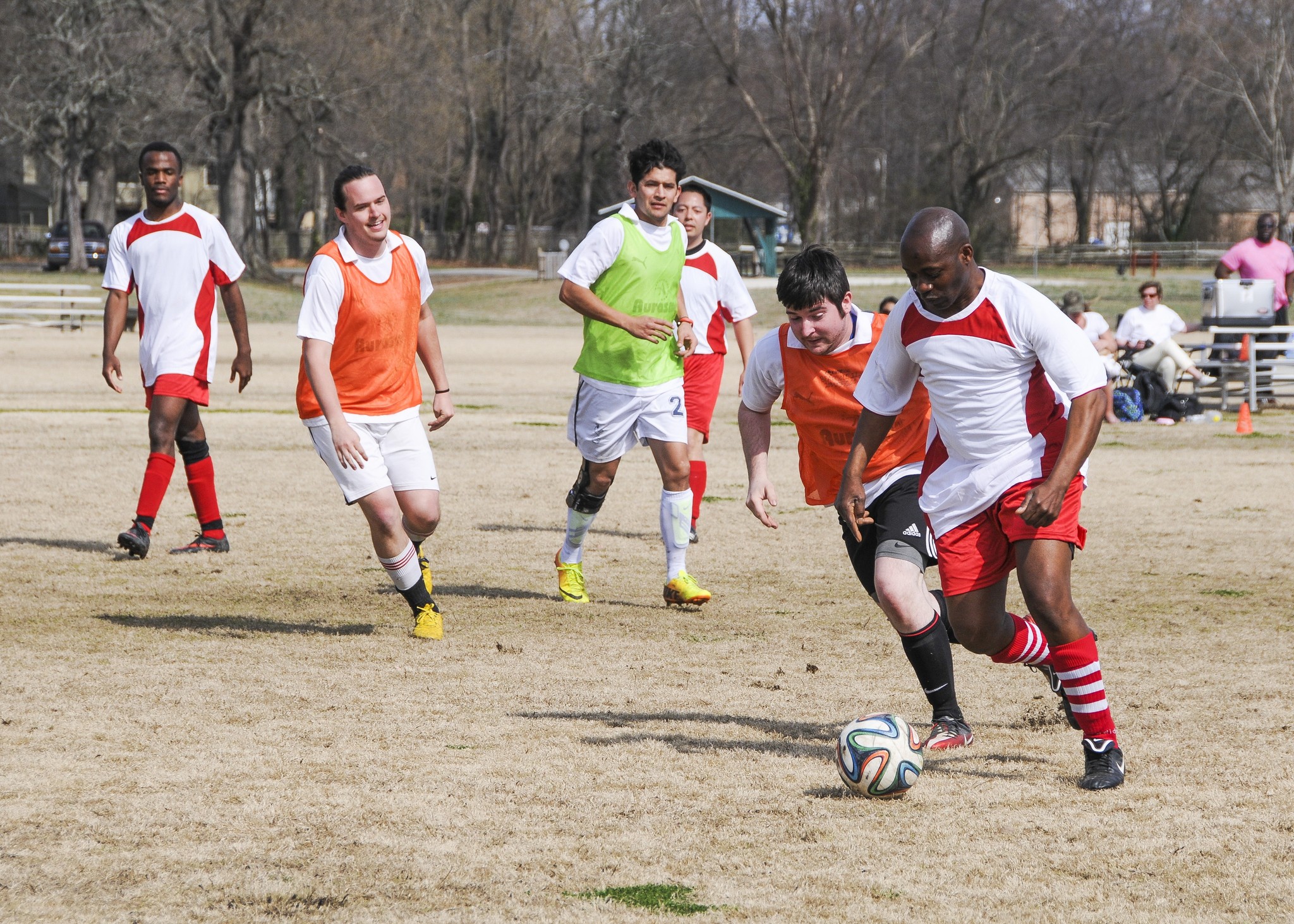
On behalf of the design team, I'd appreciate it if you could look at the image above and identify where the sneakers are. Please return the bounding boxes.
[1081,737,1125,790]
[554,548,590,603]
[1022,613,1097,730]
[168,534,229,555]
[663,570,711,606]
[1198,374,1217,388]
[415,545,433,594]
[117,522,150,559]
[923,718,973,752]
[690,527,698,543]
[412,603,444,640]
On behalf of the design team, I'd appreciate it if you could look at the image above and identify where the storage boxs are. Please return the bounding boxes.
[1200,278,1276,326]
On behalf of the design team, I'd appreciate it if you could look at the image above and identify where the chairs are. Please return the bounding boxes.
[1114,313,1206,398]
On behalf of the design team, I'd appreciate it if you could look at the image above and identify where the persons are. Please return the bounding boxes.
[1057,288,1122,426]
[553,138,714,608]
[661,183,760,545]
[1214,213,1294,359]
[1113,280,1217,394]
[835,206,1133,791]
[738,242,1062,751]
[878,295,899,316]
[101,139,256,560]
[295,165,456,642]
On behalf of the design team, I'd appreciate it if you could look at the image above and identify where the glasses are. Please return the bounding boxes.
[1141,292,1158,298]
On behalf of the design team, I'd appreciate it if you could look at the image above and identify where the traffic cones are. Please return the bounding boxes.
[1235,402,1255,435]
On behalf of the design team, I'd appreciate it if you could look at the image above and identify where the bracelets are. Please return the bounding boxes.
[677,315,693,329]
[434,388,451,394]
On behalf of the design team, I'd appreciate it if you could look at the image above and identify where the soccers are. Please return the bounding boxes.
[832,712,926,795]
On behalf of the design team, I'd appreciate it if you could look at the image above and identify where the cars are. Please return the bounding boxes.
[44,218,115,273]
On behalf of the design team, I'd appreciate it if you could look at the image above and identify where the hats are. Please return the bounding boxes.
[1060,290,1085,313]
